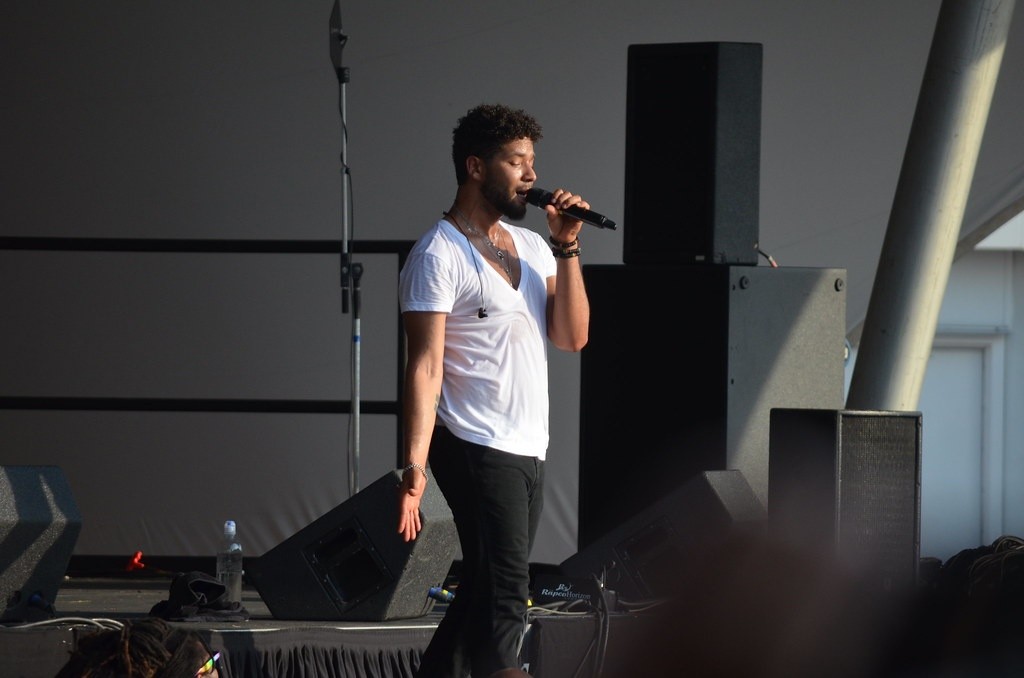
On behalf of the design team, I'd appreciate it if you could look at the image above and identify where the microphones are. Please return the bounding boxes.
[526,187,617,230]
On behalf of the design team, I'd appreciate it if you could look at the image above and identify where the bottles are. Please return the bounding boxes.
[216,520,241,603]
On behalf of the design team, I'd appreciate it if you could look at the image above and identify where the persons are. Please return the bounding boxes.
[54,617,221,678]
[395,105,590,678]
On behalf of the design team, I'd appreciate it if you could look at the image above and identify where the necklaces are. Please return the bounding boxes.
[450,202,517,291]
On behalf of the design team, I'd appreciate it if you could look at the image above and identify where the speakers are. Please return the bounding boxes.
[536,466,768,613]
[579,263,846,549]
[766,408,921,618]
[247,466,462,622]
[1,465,84,624]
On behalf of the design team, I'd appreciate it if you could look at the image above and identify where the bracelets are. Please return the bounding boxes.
[402,463,428,482]
[548,235,582,259]
